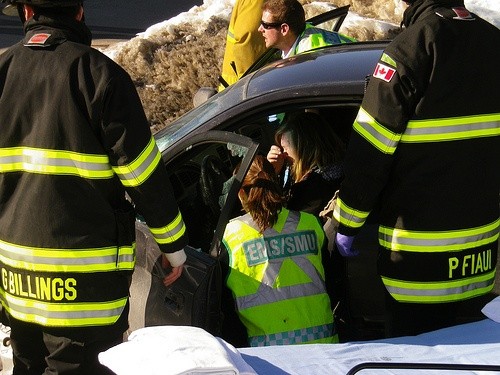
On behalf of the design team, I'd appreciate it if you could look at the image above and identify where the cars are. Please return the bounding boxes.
[125,6,499,349]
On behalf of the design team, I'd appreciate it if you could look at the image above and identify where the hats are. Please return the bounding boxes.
[2,0,83,16]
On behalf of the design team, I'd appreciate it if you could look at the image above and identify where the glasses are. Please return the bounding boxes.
[261,21,282,29]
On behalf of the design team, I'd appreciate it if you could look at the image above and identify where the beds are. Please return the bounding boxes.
[98,295,500,375]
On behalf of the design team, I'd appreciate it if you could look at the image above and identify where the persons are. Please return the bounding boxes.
[209,155,342,347]
[0,0,188,374]
[267,112,345,215]
[257,0,361,58]
[331,0,500,338]
[219,1,273,93]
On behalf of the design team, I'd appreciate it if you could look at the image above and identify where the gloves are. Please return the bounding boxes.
[334,232,362,257]
